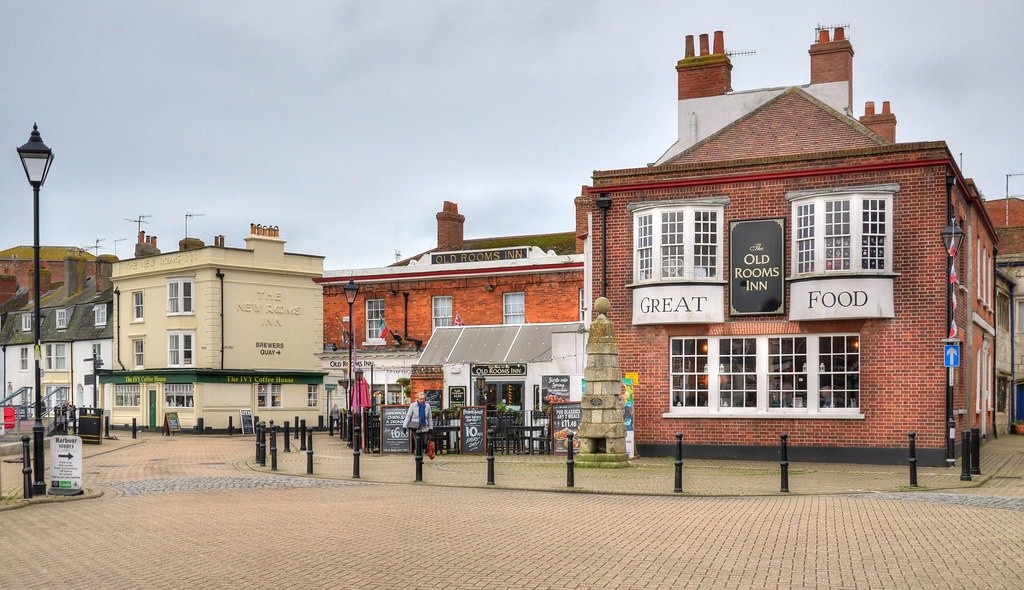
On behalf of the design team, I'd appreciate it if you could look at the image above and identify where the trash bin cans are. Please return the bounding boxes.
[78,407,103,445]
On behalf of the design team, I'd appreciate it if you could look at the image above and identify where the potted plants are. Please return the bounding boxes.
[495,402,506,416]
[430,407,441,419]
[1015,419,1024,435]
[443,405,460,420]
[396,378,410,386]
[404,387,411,397]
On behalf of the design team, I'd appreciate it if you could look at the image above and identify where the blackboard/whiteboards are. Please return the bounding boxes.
[460,406,487,455]
[379,405,411,456]
[550,402,582,455]
[165,413,182,431]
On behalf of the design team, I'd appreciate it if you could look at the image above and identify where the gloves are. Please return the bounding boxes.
[403,428,406,434]
[429,429,435,436]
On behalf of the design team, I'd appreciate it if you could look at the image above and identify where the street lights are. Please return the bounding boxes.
[16,122,55,496]
[941,216,966,467]
[342,270,359,447]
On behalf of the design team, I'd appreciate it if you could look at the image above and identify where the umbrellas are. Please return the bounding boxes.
[353,377,372,414]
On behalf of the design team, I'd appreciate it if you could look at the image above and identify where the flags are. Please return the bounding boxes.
[454,315,463,326]
[378,316,389,340]
[342,327,347,348]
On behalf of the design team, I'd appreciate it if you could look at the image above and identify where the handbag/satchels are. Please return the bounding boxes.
[427,434,436,460]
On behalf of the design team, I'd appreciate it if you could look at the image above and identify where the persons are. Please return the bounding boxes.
[331,404,340,432]
[403,392,434,464]
